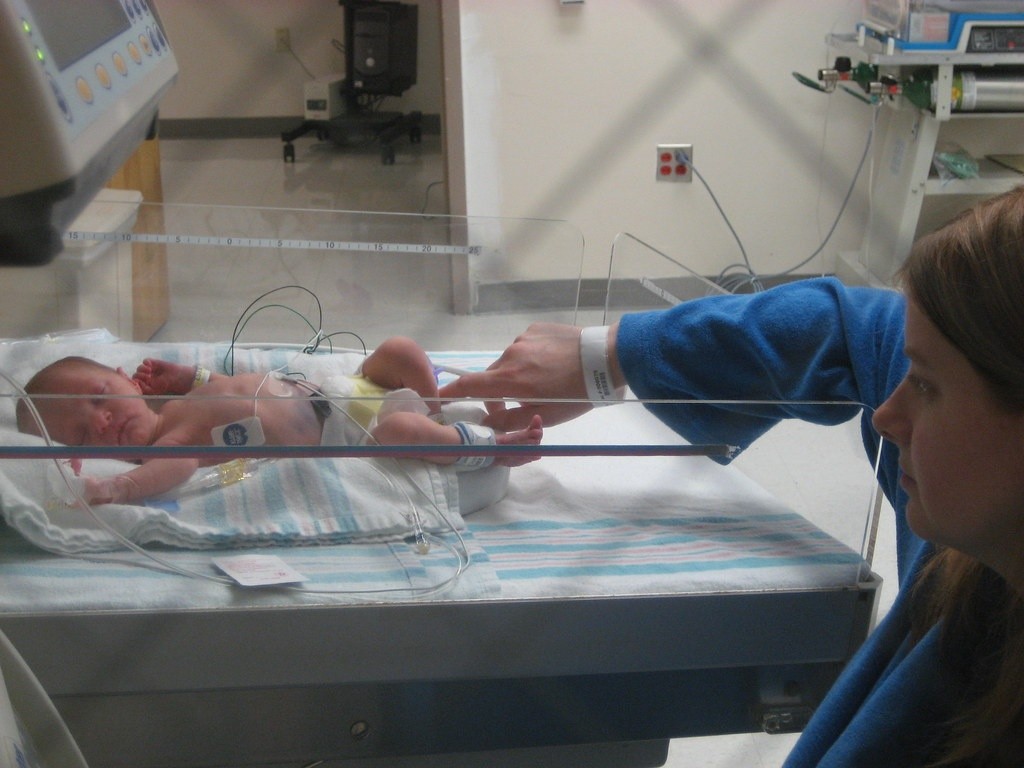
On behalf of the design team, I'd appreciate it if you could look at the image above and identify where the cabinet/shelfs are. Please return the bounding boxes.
[0,187,144,341]
[834,96,1024,288]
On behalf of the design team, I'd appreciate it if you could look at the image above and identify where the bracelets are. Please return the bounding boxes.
[192,364,210,387]
[580,326,627,408]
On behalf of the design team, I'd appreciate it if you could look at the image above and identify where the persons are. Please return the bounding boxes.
[17,335,544,507]
[439,191,1024,767]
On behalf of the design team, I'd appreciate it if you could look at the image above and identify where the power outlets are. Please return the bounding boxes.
[274,26,291,53]
[655,144,694,183]
